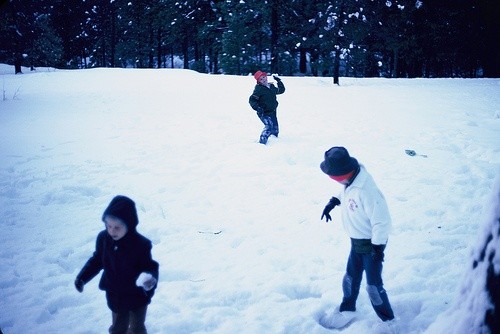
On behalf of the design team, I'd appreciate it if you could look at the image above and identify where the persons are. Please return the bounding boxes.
[319,144,400,334]
[248,71,286,144]
[72,194,159,334]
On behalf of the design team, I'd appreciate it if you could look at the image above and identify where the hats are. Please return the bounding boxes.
[254,70,266,84]
[320,146,359,176]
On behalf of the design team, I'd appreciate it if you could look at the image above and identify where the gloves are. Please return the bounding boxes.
[273,76,281,82]
[75,277,85,292]
[370,244,386,264]
[321,196,341,222]
[143,277,157,292]
